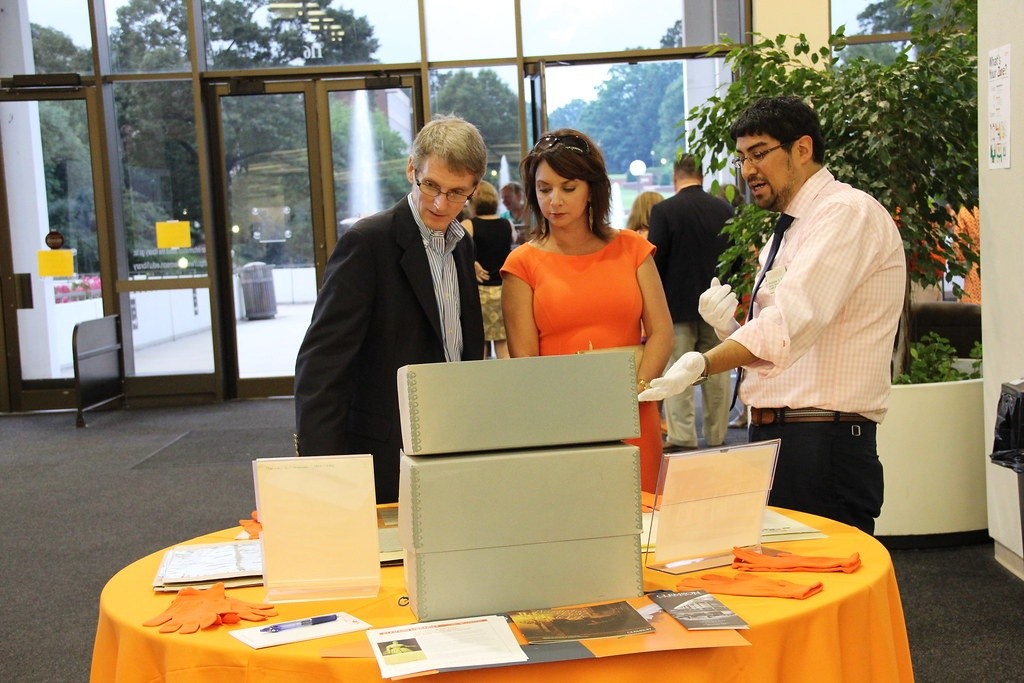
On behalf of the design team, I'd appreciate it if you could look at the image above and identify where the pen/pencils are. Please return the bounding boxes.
[260,615,337,633]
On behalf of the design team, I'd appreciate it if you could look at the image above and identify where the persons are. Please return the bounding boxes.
[637,96,906,537]
[626,153,755,456]
[294,117,486,505]
[450,180,552,361]
[499,129,675,496]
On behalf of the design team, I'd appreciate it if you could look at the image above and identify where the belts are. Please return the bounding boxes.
[751,406,866,425]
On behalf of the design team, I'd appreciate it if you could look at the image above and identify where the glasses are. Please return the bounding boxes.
[414,168,478,203]
[732,136,799,169]
[530,134,590,155]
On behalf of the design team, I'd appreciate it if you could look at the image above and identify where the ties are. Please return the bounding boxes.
[730,214,795,411]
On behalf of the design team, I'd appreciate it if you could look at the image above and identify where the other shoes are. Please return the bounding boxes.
[663,442,698,454]
[729,414,747,428]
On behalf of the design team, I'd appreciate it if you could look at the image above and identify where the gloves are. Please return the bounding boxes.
[637,351,704,402]
[143,581,278,634]
[698,277,742,341]
[731,544,861,574]
[239,511,262,539]
[676,572,823,601]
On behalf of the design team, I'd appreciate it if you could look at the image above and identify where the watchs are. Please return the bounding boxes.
[692,355,710,387]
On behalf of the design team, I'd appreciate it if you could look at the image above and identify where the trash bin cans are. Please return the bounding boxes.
[234,264,277,320]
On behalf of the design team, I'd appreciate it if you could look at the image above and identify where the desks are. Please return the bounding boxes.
[90,491,914,683]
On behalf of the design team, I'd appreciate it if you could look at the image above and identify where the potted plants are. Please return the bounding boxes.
[677,0,987,549]
